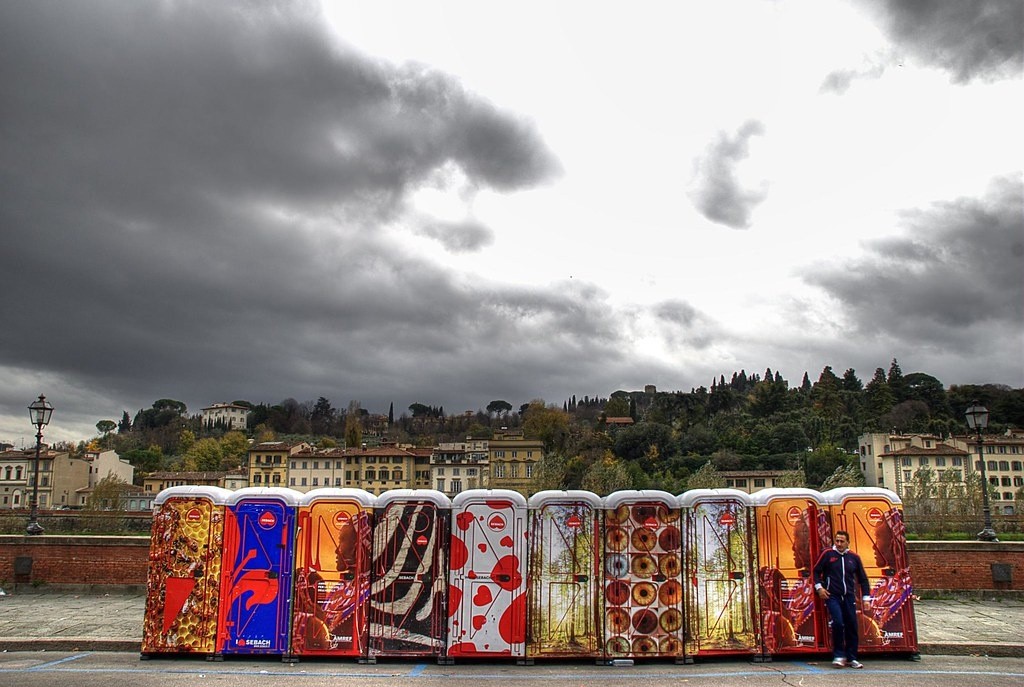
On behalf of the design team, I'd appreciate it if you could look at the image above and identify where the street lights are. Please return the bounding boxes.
[26,391,56,534]
[965,398,998,541]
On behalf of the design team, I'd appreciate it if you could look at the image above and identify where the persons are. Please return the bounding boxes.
[811,532,872,669]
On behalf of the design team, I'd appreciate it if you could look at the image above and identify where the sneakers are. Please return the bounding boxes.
[846,660,863,669]
[832,658,846,666]
